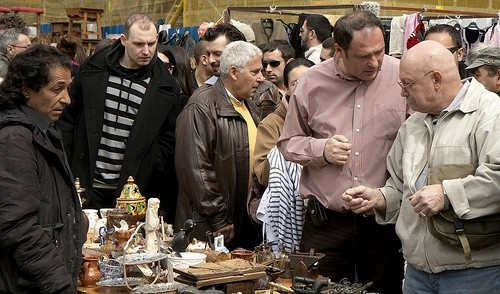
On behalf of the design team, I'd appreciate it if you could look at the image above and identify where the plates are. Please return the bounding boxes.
[96,277,146,286]
[118,253,169,265]
[131,283,187,293]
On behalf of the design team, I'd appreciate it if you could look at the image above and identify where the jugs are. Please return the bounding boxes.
[80,255,100,288]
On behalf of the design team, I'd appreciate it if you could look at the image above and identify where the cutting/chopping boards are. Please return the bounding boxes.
[173,257,266,289]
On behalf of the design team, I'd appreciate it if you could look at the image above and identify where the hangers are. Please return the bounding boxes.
[251,9,292,30]
[160,25,195,47]
[382,17,391,30]
[418,17,500,39]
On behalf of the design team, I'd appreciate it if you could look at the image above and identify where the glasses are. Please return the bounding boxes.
[165,63,174,70]
[447,47,459,54]
[397,69,442,89]
[300,28,311,32]
[264,60,286,68]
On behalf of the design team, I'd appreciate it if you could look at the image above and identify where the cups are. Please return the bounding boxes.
[98,260,125,278]
[81,208,98,233]
[100,208,114,224]
[231,249,254,262]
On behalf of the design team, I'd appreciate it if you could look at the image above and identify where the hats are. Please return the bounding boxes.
[465,46,500,72]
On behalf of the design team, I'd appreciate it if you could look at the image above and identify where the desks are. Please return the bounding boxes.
[76,232,365,294]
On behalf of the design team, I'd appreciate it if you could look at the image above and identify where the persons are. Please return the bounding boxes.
[175,41,266,252]
[342,40,500,294]
[0,14,500,253]
[278,10,416,294]
[0,44,87,294]
[58,13,181,210]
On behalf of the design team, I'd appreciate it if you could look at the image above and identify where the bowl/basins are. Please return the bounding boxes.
[261,265,286,282]
[168,252,207,270]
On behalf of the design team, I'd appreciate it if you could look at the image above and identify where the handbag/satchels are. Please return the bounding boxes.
[426,163,500,247]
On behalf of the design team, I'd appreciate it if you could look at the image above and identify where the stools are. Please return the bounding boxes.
[65,7,104,39]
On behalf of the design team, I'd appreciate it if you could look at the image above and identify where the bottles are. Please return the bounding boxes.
[277,255,319,280]
[101,229,115,256]
